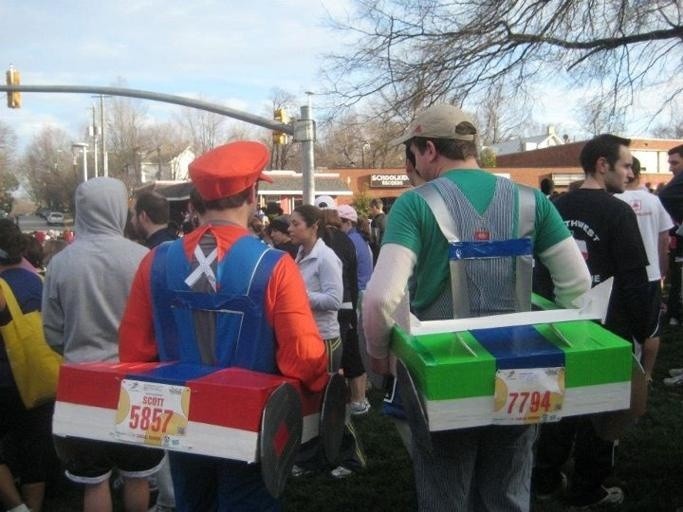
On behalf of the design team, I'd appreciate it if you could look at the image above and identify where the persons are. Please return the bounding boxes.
[533,134,651,512]
[41,176,166,512]
[118,141,330,512]
[1,215,74,511]
[128,144,683,512]
[363,103,593,512]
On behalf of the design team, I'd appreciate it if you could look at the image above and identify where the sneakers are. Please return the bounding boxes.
[570,485,623,512]
[291,398,370,477]
[663,368,682,387]
[536,473,566,500]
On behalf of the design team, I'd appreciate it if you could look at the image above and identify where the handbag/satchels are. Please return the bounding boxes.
[0,311,63,410]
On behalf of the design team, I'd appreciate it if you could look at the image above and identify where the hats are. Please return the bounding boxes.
[188,141,272,201]
[336,205,357,223]
[314,195,336,211]
[391,105,477,145]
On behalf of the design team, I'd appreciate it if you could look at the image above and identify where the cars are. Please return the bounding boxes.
[46,211,66,225]
